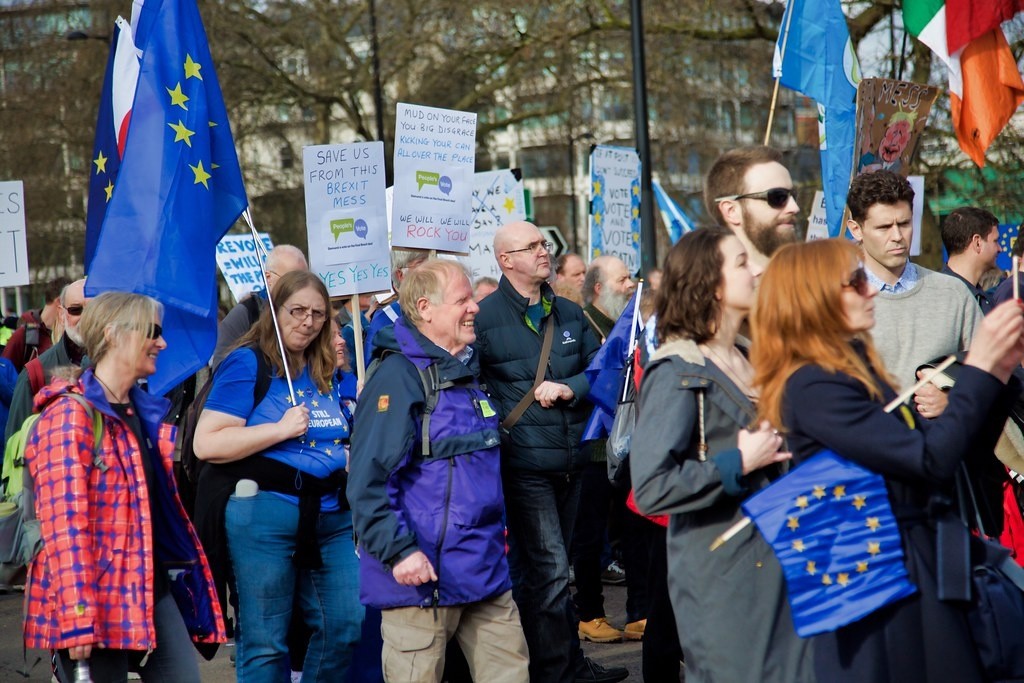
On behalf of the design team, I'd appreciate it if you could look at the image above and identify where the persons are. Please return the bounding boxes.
[0,129,1024,683]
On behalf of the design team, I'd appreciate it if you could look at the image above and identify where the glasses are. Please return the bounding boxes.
[505,242,554,253]
[62,305,83,315]
[715,185,798,208]
[841,268,868,295]
[125,323,162,340]
[280,305,329,322]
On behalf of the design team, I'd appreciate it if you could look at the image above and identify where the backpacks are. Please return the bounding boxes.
[181,341,271,485]
[0,393,105,564]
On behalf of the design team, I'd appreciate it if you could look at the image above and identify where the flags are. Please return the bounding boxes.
[769,0,865,246]
[742,451,916,637]
[86,0,250,399]
[581,290,637,442]
[651,181,693,244]
[901,0,1024,168]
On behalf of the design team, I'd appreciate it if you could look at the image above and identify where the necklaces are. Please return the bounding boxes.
[706,342,762,400]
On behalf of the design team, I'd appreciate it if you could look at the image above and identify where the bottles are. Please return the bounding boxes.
[74,658,93,683]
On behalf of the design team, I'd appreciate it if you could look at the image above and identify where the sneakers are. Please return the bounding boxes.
[576,657,629,683]
[604,563,628,583]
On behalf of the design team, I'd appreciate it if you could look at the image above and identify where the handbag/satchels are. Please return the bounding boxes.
[933,458,1024,683]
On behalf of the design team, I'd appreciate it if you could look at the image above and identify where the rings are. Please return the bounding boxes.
[922,405,927,412]
[771,428,780,435]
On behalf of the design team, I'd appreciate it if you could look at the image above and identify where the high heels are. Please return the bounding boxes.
[578,617,623,642]
[623,616,646,640]
